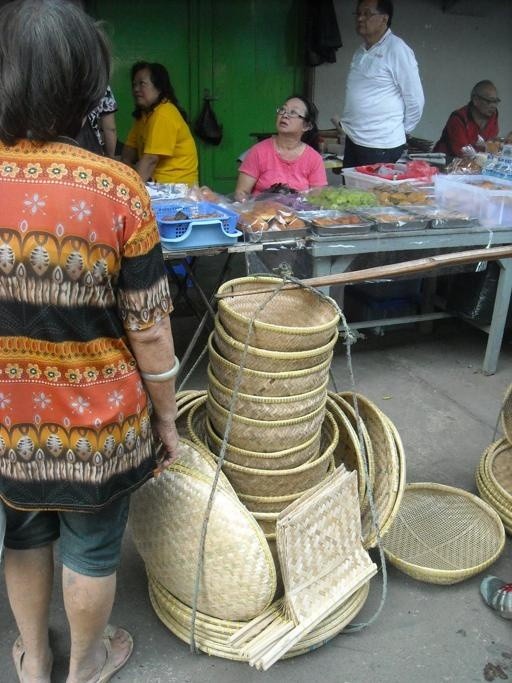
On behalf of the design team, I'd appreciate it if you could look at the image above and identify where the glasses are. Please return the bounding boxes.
[354,11,384,20]
[477,94,503,107]
[276,104,311,123]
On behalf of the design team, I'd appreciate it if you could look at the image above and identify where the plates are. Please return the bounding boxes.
[398,204,477,229]
[296,208,374,236]
[346,205,432,231]
[236,214,312,243]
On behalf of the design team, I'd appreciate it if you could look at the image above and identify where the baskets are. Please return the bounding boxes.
[263,533,285,602]
[215,275,341,351]
[203,386,328,453]
[249,512,280,536]
[232,455,335,512]
[207,363,329,421]
[140,584,370,661]
[207,331,334,397]
[187,397,340,497]
[175,437,238,498]
[125,458,277,622]
[173,393,207,429]
[500,383,512,446]
[323,398,366,508]
[374,481,506,586]
[204,416,323,469]
[363,398,408,550]
[475,435,512,539]
[177,389,206,409]
[212,313,339,371]
[173,390,195,401]
[336,390,399,544]
[322,390,377,518]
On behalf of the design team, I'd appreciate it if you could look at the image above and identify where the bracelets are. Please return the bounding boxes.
[141,356,180,382]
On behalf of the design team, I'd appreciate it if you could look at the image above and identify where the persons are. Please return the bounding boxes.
[86,84,118,161]
[120,61,199,190]
[233,94,328,202]
[1,0,179,681]
[341,0,425,185]
[431,80,500,164]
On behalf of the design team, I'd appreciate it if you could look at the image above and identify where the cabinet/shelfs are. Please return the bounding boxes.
[147,170,512,374]
[250,127,433,169]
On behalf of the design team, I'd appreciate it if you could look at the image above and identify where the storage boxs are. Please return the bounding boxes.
[342,164,425,192]
[150,202,239,238]
[432,174,512,228]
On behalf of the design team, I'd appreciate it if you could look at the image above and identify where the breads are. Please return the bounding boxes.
[307,212,366,229]
[369,180,437,209]
[225,198,304,237]
[187,183,222,206]
[297,185,377,210]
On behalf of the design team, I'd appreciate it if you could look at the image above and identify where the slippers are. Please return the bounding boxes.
[11,632,54,683]
[65,625,134,683]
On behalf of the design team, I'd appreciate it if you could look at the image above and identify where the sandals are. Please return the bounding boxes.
[480,575,511,619]
